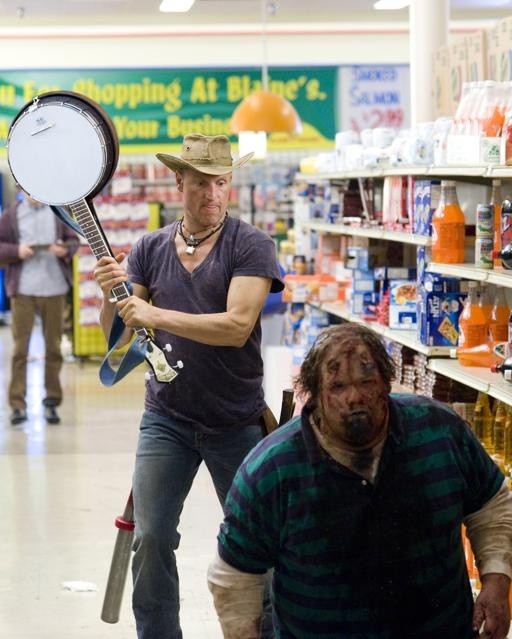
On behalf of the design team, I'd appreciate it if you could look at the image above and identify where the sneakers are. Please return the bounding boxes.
[9,409,29,427]
[42,406,61,424]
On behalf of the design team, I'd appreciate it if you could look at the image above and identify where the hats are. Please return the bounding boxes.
[154,130,257,178]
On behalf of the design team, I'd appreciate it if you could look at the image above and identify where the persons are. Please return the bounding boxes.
[206,320,512,638]
[1,192,79,425]
[92,133,284,638]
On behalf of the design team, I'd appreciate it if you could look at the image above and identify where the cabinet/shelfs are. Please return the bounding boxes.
[293,165,512,407]
[65,152,292,360]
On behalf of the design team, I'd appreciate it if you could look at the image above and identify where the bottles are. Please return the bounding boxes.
[454,79,512,166]
[471,390,512,493]
[430,179,467,264]
[489,179,504,269]
[489,283,511,343]
[457,281,488,368]
[449,343,512,384]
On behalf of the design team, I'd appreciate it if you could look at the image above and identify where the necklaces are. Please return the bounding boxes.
[176,211,228,255]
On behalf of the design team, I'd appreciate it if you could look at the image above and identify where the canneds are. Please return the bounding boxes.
[474,239,495,270]
[477,202,495,238]
